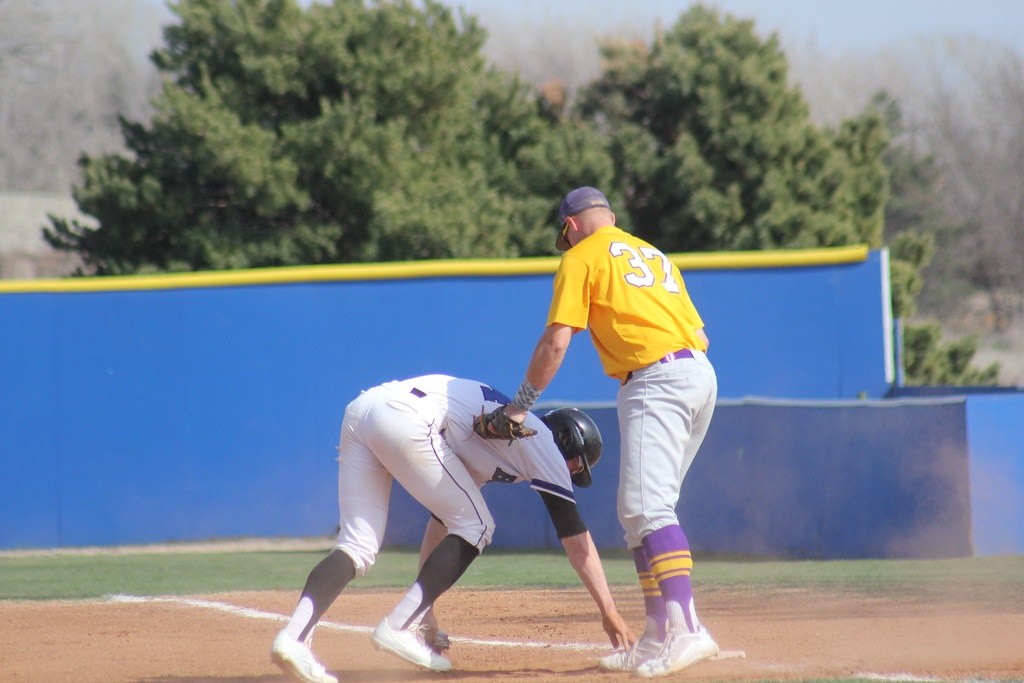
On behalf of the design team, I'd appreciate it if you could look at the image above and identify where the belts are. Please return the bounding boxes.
[410,387,444,437]
[628,348,694,380]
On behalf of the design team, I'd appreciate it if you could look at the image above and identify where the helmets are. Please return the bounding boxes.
[545,407,602,487]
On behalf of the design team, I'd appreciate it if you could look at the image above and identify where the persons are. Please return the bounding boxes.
[272,374,636,683]
[472,187,720,678]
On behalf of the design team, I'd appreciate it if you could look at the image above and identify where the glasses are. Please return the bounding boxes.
[562,222,569,242]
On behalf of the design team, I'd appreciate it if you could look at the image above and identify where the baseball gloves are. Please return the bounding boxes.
[472,405,539,441]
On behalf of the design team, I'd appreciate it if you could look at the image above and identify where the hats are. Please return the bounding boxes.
[555,187,610,252]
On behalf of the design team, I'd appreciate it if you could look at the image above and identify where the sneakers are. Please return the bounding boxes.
[639,625,719,679]
[598,635,667,672]
[271,629,338,683]
[371,617,451,672]
